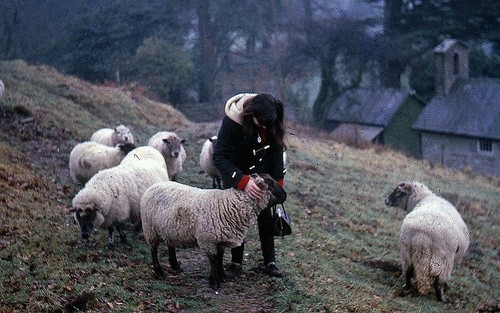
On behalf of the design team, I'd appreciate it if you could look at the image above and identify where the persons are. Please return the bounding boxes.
[212,93,291,278]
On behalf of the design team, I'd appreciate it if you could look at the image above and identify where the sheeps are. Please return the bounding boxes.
[69,140,137,186]
[90,125,135,147]
[147,130,187,182]
[140,172,287,292]
[198,135,287,189]
[118,146,167,169]
[385,180,470,302]
[69,159,170,250]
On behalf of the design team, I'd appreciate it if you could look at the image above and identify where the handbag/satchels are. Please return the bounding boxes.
[273,203,292,236]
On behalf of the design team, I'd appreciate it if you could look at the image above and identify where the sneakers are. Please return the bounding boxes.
[266,264,284,278]
[225,264,242,276]
